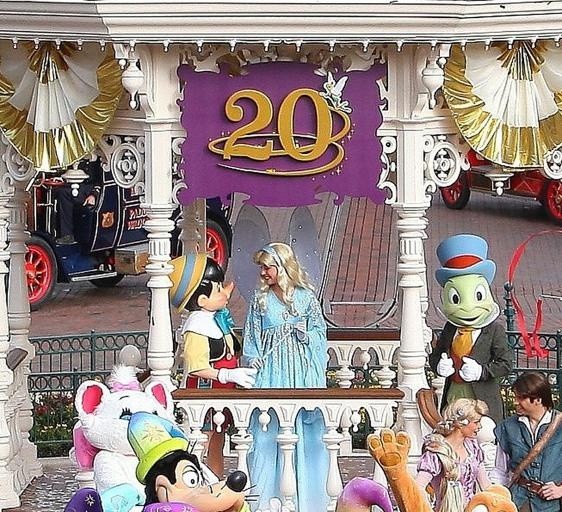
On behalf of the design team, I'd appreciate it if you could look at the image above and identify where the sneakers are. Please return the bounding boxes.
[56,235,75,244]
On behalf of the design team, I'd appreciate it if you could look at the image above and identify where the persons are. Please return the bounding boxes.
[37,150,102,245]
[168,253,258,389]
[414,395,488,512]
[428,235,513,426]
[240,243,330,388]
[488,370,562,512]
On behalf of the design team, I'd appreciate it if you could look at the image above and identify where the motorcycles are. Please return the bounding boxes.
[5,94,232,313]
[436,93,561,222]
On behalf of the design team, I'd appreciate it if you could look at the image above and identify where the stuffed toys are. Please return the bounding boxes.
[128,411,393,512]
[64,367,181,512]
[367,429,517,512]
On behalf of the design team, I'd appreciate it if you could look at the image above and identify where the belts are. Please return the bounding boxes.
[515,474,561,494]
